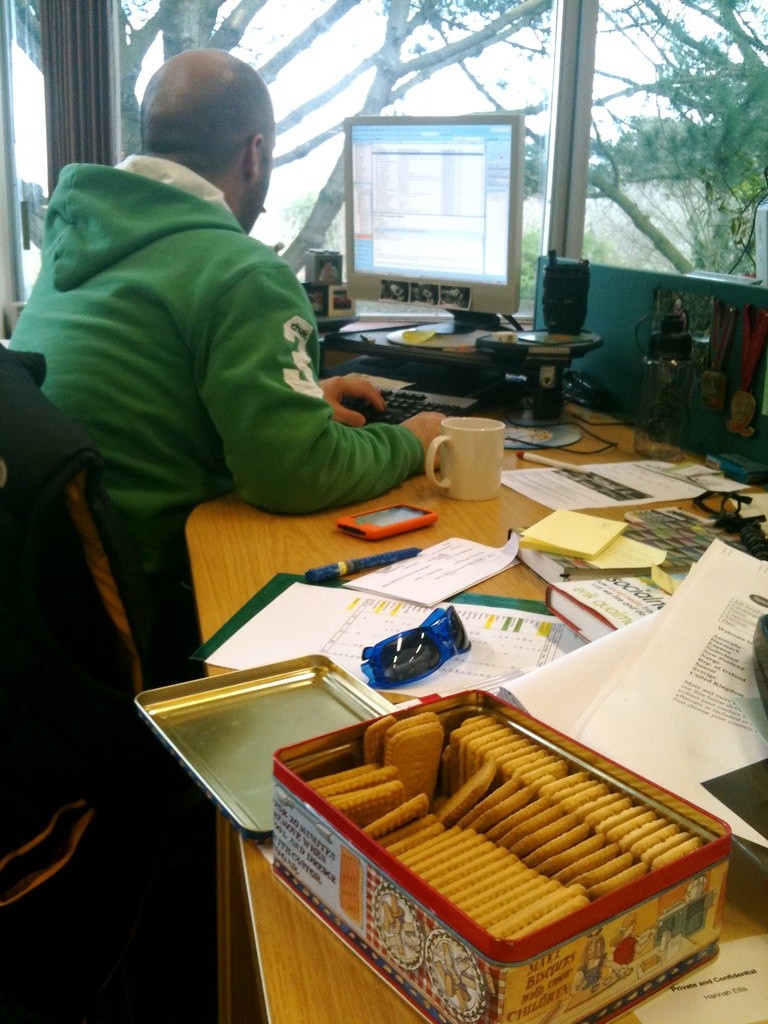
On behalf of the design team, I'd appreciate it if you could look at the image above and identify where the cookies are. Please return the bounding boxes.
[301,710,708,940]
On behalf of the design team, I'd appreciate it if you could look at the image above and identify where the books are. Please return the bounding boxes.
[508,523,749,644]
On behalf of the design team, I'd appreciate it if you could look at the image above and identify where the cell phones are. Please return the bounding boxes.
[337,504,430,540]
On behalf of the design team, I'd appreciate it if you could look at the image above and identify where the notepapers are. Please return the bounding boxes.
[518,508,629,561]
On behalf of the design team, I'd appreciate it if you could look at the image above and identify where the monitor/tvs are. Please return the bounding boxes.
[344,114,526,348]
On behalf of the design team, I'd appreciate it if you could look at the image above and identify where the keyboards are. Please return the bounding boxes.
[343,388,478,426]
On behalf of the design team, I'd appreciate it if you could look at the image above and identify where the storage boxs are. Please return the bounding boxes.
[134,653,732,1024]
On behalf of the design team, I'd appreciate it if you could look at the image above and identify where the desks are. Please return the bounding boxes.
[186,403,768,1024]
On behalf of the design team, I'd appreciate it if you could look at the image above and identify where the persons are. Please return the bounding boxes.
[0,348,163,1024]
[8,51,450,602]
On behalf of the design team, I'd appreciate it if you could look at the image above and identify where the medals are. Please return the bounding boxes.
[725,390,756,437]
[701,370,726,409]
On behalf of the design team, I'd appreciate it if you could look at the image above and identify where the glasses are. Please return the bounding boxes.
[360,606,472,688]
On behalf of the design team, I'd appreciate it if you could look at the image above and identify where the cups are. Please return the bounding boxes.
[634,314,696,458]
[542,265,590,335]
[425,417,506,501]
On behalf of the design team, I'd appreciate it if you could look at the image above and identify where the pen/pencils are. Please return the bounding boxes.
[516,451,591,475]
[304,546,422,584]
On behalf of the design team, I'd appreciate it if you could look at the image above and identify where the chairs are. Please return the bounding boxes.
[49,468,147,711]
